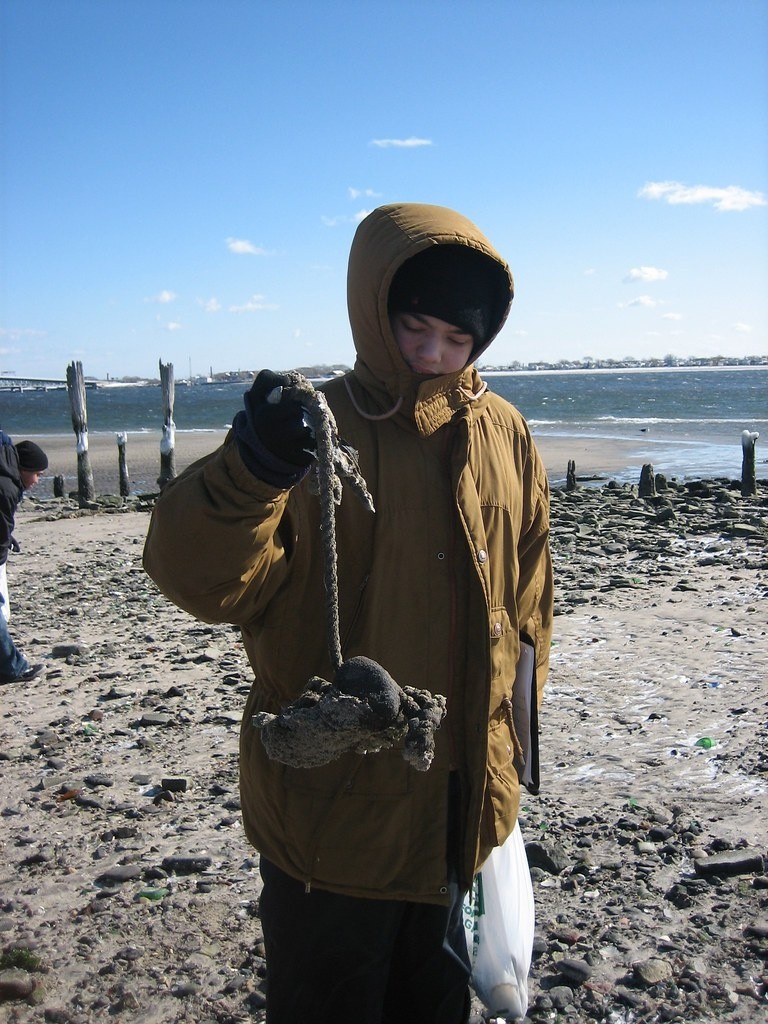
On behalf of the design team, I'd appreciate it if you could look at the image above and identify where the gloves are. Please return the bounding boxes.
[249,370,317,463]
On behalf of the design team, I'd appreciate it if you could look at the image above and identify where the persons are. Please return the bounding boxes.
[0,428,48,686]
[140,202,555,1023]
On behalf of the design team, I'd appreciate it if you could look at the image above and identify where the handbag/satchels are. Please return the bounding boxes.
[463,819,535,1021]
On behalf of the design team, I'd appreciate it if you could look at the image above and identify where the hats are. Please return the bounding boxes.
[15,440,48,472]
[388,245,490,350]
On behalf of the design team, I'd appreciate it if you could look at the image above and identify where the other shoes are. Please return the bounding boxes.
[12,663,46,682]
